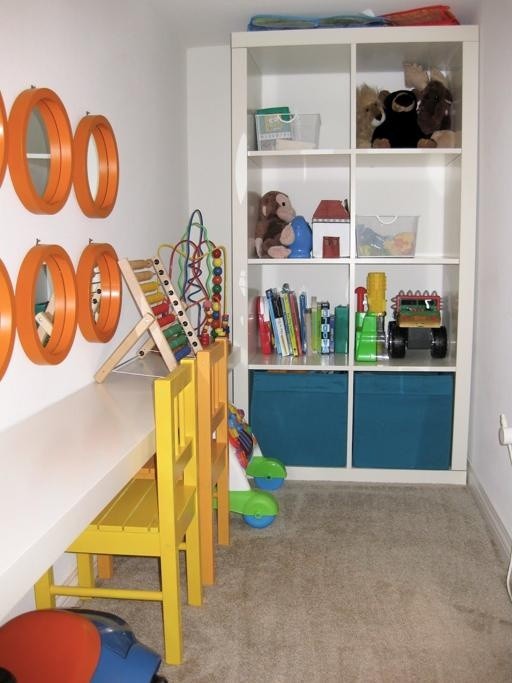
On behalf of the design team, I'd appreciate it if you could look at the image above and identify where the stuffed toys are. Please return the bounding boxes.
[254,190,297,260]
[371,88,438,149]
[402,60,457,149]
[356,83,386,149]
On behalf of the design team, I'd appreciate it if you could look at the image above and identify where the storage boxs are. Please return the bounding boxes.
[355,214,419,258]
[254,112,321,150]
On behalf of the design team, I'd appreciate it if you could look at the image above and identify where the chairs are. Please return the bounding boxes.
[98,336,230,586]
[33,356,203,666]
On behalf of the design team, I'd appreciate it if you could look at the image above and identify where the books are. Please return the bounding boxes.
[256,283,349,357]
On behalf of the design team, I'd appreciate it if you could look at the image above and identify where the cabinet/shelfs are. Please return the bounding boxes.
[231,26,469,490]
